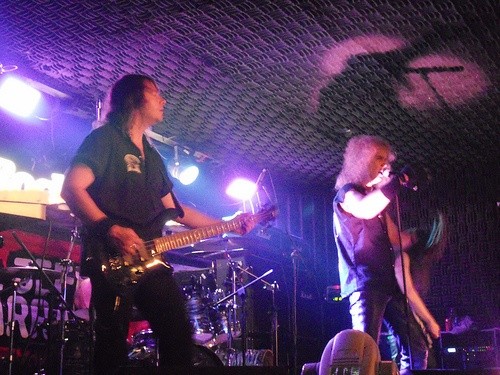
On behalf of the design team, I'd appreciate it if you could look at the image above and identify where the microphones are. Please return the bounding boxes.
[250,169,267,199]
[401,180,418,192]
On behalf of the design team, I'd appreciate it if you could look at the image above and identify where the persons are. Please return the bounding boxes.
[61,74,258,375]
[330,136,439,375]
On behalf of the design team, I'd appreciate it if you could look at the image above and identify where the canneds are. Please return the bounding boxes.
[445,317,453,331]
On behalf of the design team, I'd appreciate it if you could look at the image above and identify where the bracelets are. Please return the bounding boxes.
[94,217,116,240]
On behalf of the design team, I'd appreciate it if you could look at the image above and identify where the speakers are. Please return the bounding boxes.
[47,320,94,375]
[321,301,352,351]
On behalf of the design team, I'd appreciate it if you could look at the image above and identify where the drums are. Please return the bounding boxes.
[135,331,225,367]
[229,347,272,366]
[46,321,92,368]
[186,290,243,347]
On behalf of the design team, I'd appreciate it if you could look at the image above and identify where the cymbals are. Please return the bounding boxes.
[183,233,250,261]
[0,265,62,280]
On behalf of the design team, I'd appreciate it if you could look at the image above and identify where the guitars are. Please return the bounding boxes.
[90,208,272,296]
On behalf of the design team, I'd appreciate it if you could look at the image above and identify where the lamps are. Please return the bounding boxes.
[166,146,199,185]
